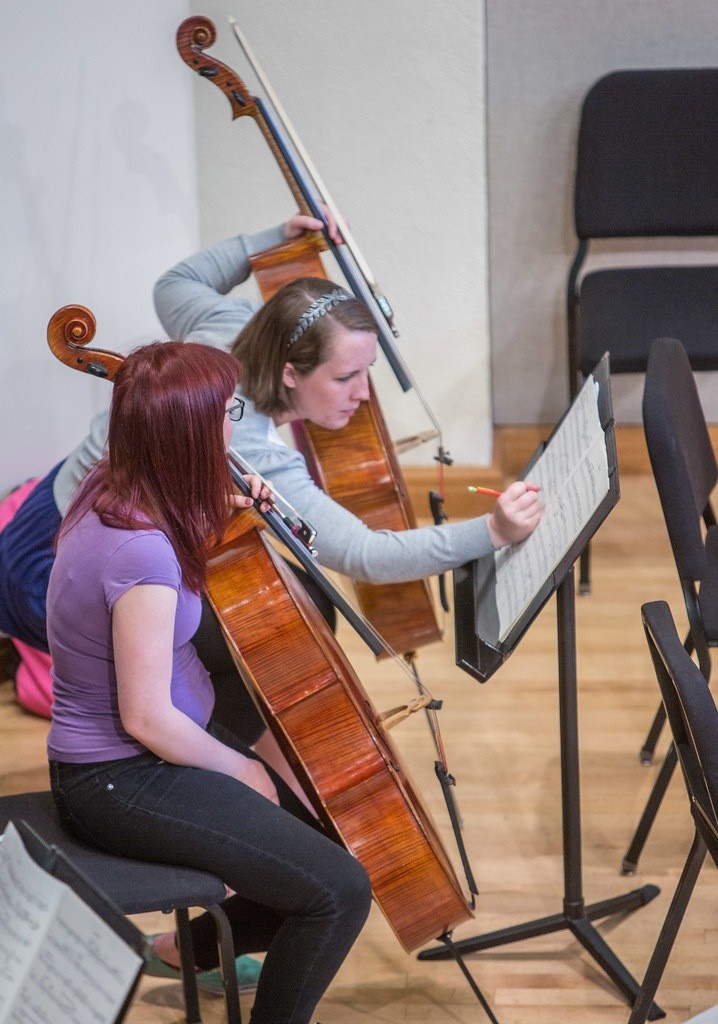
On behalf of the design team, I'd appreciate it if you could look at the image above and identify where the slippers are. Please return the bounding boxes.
[140,931,262,995]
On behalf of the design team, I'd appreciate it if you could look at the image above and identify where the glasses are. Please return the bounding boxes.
[224,395,245,422]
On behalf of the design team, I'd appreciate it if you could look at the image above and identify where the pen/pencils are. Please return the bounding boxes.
[469,483,501,502]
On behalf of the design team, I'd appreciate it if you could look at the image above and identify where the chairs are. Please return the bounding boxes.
[566,68,718,593]
[619,599,718,1023]
[638,336,718,765]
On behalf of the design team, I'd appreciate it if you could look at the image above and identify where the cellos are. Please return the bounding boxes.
[168,13,446,657]
[47,304,474,959]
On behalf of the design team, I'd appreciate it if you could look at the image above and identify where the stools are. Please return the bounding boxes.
[0,789,244,1024]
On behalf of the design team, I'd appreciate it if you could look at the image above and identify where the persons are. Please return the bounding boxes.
[0,202,543,751]
[49,343,372,1024]
